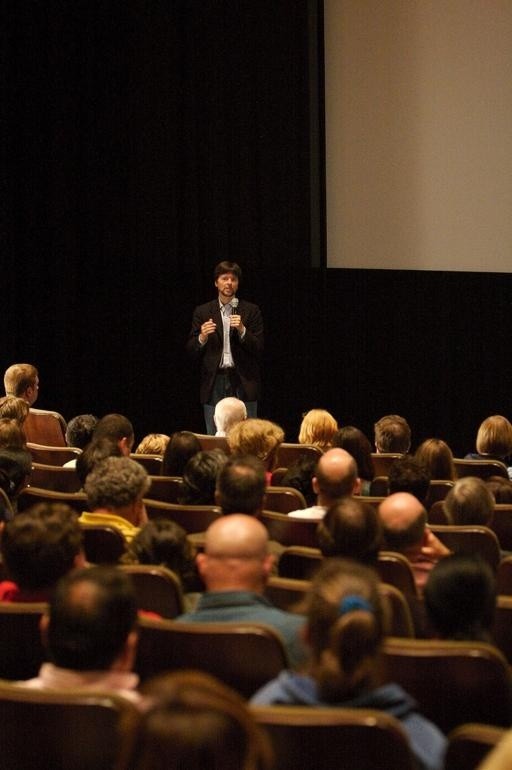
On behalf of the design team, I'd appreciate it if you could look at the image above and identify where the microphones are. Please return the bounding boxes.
[230,297,239,338]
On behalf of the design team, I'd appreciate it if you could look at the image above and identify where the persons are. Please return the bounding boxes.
[188,260,267,436]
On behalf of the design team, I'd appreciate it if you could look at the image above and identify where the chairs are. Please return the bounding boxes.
[1,433,512,769]
[20,407,67,447]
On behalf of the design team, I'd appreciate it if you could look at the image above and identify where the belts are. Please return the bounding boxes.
[217,366,236,375]
